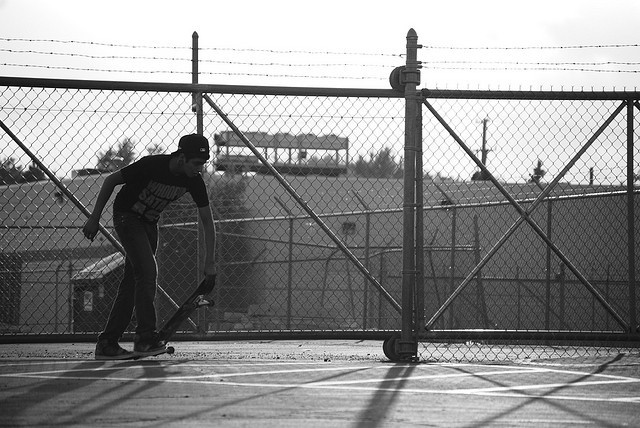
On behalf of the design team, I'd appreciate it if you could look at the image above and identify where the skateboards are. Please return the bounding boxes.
[133,274,217,360]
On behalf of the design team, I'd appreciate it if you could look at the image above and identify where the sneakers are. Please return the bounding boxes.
[95,337,134,360]
[134,331,167,357]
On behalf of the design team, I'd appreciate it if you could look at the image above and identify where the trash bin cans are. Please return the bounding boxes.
[70,247,137,332]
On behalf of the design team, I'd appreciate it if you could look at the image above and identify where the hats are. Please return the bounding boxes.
[171,133,210,160]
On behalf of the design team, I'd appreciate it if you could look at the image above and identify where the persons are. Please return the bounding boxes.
[81,133,217,359]
